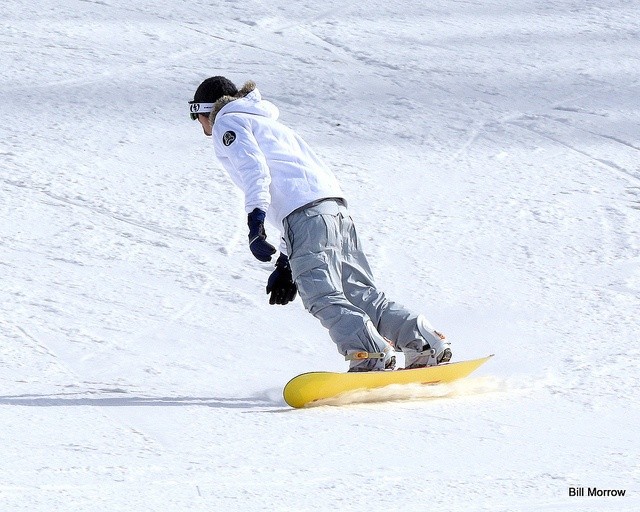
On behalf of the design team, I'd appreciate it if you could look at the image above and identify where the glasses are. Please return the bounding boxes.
[188,100,201,121]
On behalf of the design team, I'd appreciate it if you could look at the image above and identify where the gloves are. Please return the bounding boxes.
[266,257,298,306]
[248,209,275,262]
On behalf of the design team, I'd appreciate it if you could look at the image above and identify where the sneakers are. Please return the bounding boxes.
[343,321,398,371]
[395,317,452,371]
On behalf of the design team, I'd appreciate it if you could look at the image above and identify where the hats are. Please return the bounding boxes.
[192,76,238,119]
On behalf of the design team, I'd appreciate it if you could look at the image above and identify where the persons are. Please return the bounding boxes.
[188,76,452,371]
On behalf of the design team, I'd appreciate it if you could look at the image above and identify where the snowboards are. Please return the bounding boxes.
[283,353,498,408]
